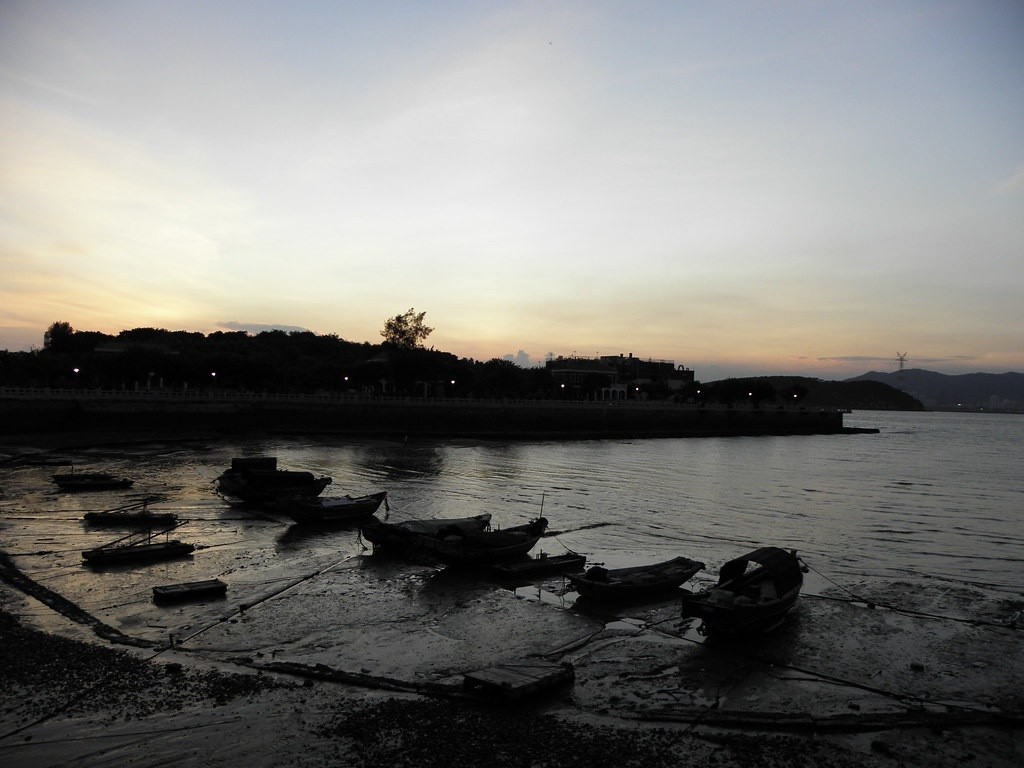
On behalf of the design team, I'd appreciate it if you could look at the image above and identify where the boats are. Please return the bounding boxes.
[47,463,134,491]
[561,553,706,603]
[228,456,332,503]
[679,544,804,636]
[83,499,178,525]
[82,513,195,563]
[274,489,388,524]
[358,492,549,562]
